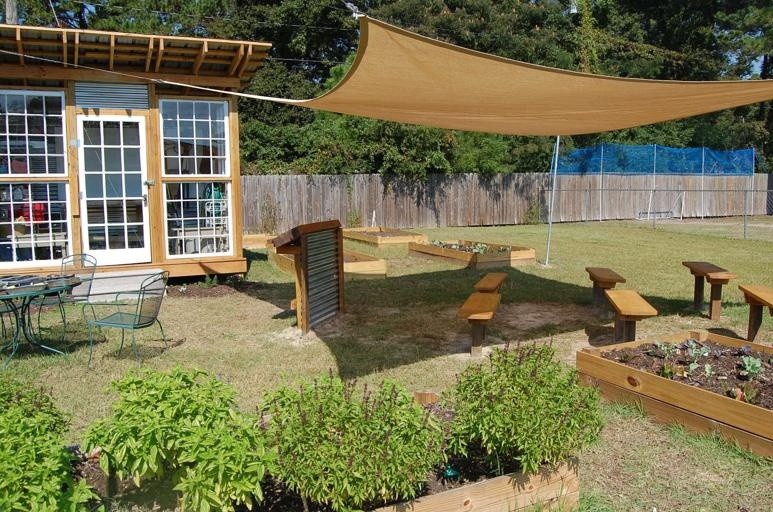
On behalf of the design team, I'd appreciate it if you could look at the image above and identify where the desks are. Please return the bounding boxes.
[0,274,80,368]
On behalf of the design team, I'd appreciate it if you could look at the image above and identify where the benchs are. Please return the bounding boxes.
[585,266,658,343]
[457,272,509,357]
[683,259,773,342]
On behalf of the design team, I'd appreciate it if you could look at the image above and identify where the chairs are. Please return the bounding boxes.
[28,252,105,349]
[84,270,170,368]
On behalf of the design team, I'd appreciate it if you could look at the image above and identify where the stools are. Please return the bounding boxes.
[1,304,24,339]
